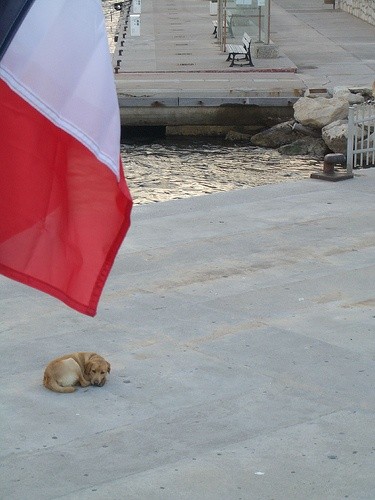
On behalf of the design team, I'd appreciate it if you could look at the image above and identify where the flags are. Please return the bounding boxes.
[0,0,134,320]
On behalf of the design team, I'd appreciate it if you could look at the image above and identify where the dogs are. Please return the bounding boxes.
[42,351,111,393]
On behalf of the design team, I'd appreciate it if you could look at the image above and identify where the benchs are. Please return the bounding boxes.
[212,9,235,38]
[225,32,254,67]
